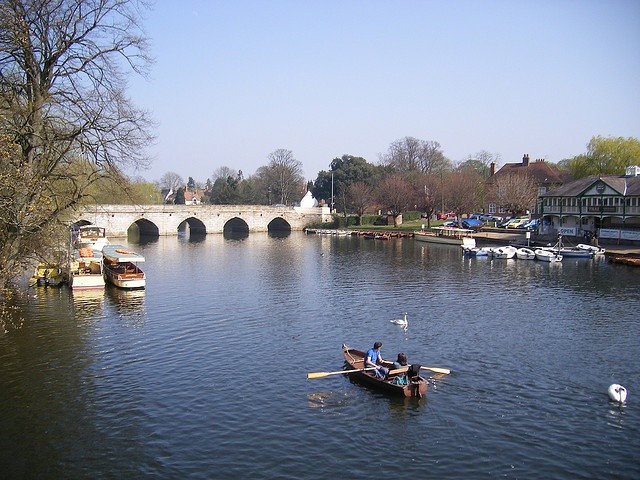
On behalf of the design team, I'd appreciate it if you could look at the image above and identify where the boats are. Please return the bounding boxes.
[35,259,62,286]
[342,343,429,400]
[464,247,494,256]
[77,225,110,250]
[517,248,535,260]
[494,246,516,259]
[535,249,556,262]
[551,244,606,258]
[103,245,146,288]
[68,249,105,290]
[413,227,476,245]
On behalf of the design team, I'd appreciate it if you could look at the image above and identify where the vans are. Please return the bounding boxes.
[508,219,529,228]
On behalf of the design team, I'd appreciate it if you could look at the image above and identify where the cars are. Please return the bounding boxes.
[437,212,447,220]
[497,219,515,228]
[421,213,428,219]
[471,213,503,222]
[522,219,539,230]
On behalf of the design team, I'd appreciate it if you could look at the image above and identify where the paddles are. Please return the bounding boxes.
[305,365,380,380]
[383,359,451,376]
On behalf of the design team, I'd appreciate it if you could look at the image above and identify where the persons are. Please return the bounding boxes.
[389,352,408,384]
[364,342,389,381]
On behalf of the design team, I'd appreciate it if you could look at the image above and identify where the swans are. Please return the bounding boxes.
[389,312,408,324]
[607,382,628,404]
